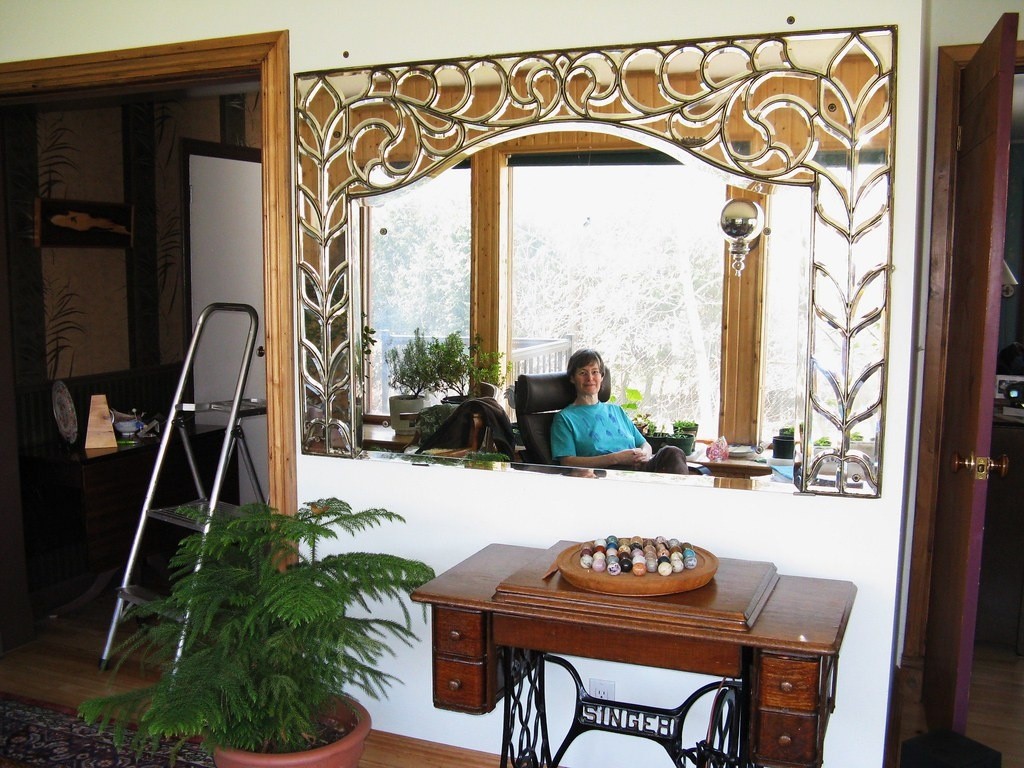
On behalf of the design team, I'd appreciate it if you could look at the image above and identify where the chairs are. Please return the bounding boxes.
[514,365,611,467]
[398,382,500,459]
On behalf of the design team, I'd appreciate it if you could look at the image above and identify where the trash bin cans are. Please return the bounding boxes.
[773,436,794,459]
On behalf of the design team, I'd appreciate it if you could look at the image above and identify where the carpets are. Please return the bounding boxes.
[0,691,216,768]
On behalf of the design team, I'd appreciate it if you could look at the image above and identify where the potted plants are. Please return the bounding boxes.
[851,432,864,441]
[667,427,695,456]
[78,497,434,768]
[673,419,699,451]
[779,424,794,436]
[773,436,794,459]
[814,436,832,446]
[632,413,652,433]
[384,325,466,438]
[440,333,513,408]
[645,421,669,454]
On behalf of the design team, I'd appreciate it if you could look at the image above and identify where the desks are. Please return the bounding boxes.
[411,544,855,768]
[19,424,240,603]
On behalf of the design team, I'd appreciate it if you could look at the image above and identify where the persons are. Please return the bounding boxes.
[550,348,689,475]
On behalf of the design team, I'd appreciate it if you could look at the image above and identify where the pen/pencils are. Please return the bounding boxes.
[117,441,138,444]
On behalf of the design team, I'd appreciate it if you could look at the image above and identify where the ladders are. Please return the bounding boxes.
[97,302,267,690]
